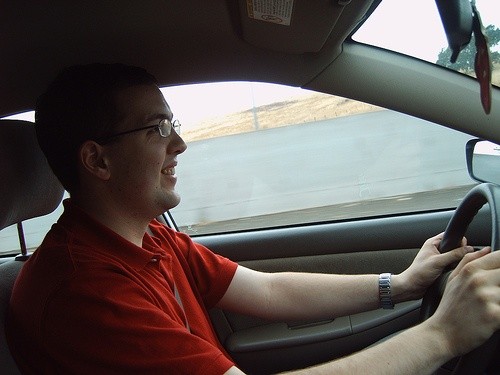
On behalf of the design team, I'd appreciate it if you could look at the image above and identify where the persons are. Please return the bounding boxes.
[5,64,500,375]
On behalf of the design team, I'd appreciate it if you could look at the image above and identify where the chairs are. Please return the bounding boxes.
[0,116,64,375]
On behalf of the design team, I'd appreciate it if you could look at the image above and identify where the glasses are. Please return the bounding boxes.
[100,118,182,142]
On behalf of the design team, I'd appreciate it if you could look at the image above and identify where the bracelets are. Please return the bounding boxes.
[379,273,395,309]
